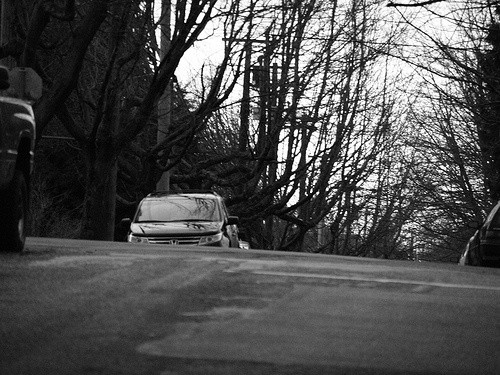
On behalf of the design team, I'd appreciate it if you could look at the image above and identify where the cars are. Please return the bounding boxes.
[457,200,500,269]
[120,187,240,249]
[0,66,37,254]
